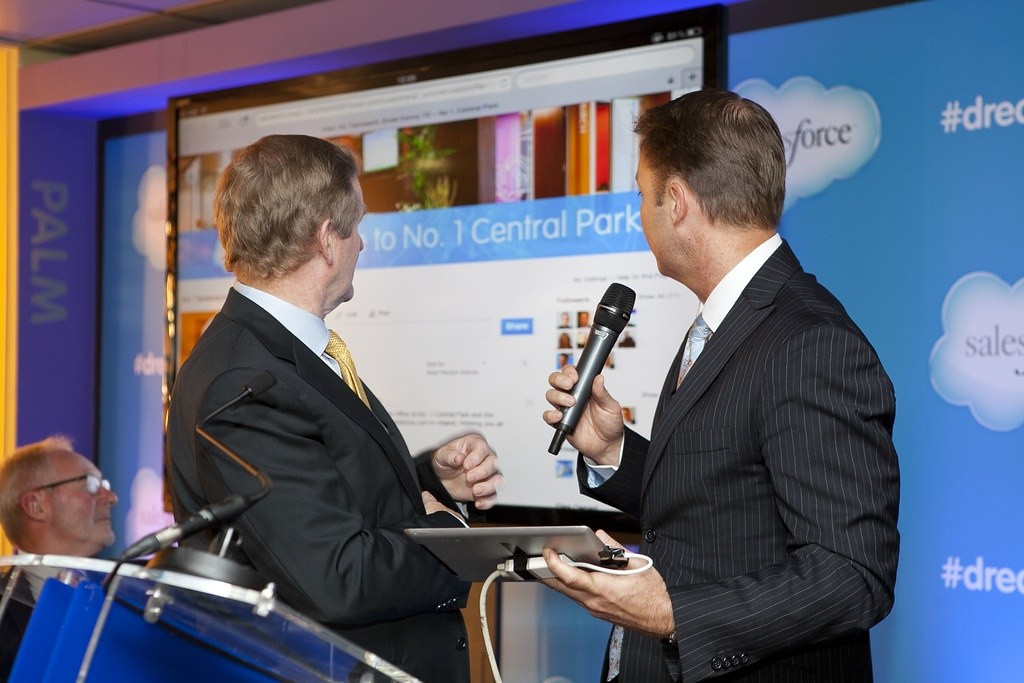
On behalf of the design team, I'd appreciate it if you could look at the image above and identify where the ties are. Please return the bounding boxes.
[325,329,372,412]
[606,313,712,682]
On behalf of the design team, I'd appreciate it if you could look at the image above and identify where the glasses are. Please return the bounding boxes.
[32,474,111,495]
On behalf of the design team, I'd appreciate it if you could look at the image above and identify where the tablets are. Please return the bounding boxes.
[405,526,623,582]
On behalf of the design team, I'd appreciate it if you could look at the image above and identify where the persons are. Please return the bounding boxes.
[163,135,503,682]
[0,437,118,682]
[542,89,900,683]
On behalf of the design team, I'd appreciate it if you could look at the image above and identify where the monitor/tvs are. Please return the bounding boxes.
[165,4,728,532]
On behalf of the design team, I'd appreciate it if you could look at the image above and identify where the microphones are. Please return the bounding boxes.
[547,282,636,457]
[118,370,278,562]
